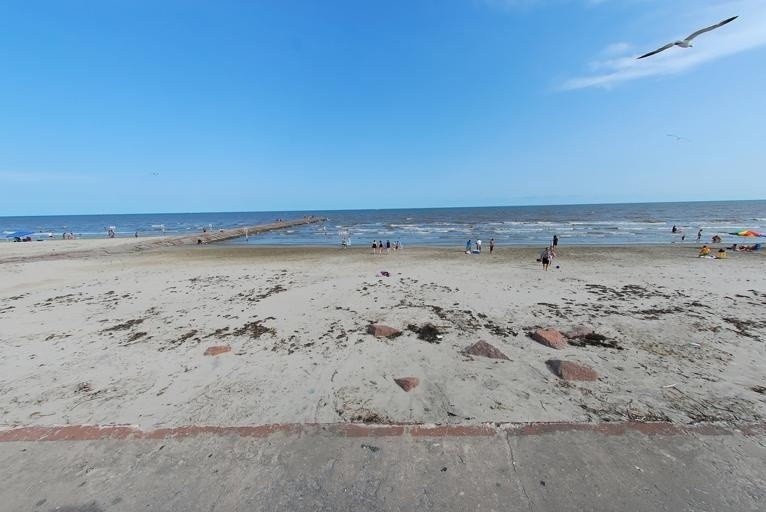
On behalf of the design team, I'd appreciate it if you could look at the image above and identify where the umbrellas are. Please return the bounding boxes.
[729,229,766,238]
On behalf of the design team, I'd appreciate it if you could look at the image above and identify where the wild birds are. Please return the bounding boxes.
[636,15,740,60]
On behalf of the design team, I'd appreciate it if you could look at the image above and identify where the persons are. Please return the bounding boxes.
[13,229,74,243]
[386,239,391,253]
[547,246,556,266]
[391,240,401,252]
[475,239,483,251]
[537,246,550,271]
[465,240,472,253]
[378,240,384,256]
[717,248,727,259]
[697,245,710,258]
[489,238,495,254]
[696,229,703,241]
[672,225,677,233]
[552,235,559,249]
[104,227,115,238]
[372,240,377,256]
[712,234,761,254]
[341,235,353,249]
